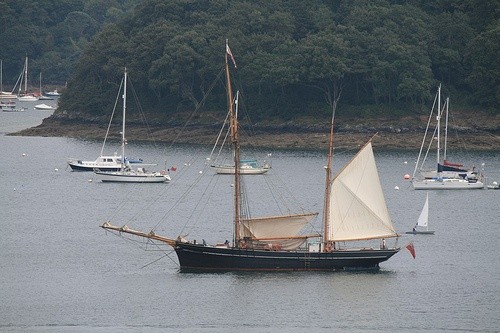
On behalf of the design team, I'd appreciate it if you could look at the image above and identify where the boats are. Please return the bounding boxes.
[47,89,63,97]
[33,104,58,111]
[487,181,500,190]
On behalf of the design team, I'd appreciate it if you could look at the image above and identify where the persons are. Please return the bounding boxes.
[381,238,386,250]
[224,240,230,244]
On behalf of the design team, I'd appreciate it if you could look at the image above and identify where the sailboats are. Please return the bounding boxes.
[210,90,271,174]
[36,72,55,100]
[66,68,158,172]
[407,82,487,190]
[97,38,419,273]
[95,66,180,182]
[407,191,444,236]
[0,52,38,112]
